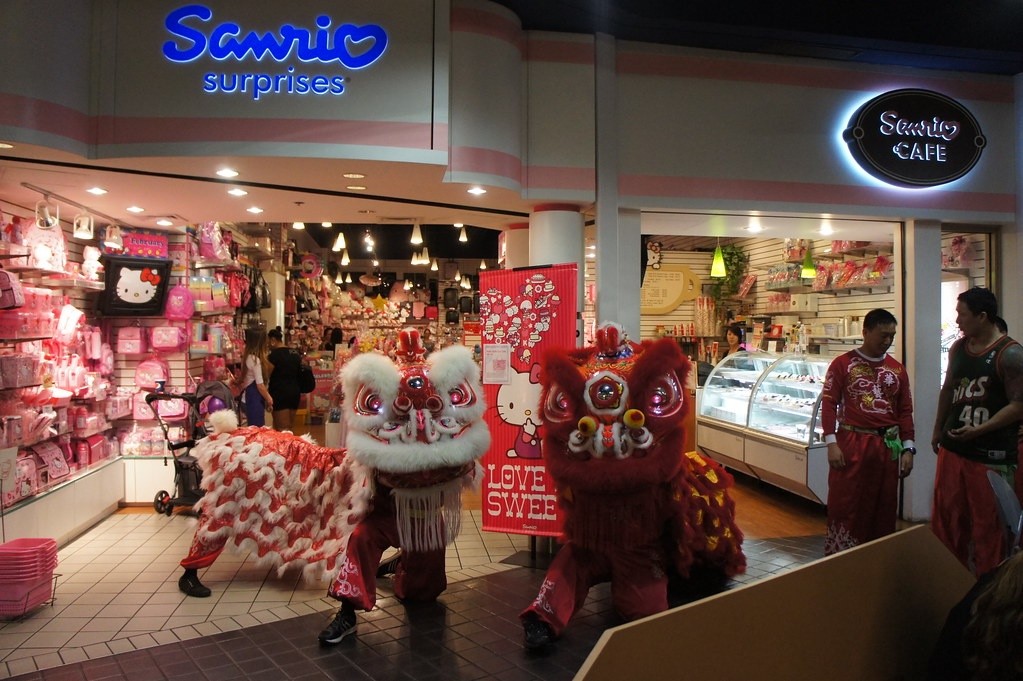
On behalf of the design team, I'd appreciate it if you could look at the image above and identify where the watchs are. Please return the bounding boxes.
[903,448,916,455]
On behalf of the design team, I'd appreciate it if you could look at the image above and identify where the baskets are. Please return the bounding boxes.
[0,537,58,619]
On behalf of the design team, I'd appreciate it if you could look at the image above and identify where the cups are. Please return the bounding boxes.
[672,295,724,367]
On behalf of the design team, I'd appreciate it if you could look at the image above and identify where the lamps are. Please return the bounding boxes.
[73,209,94,240]
[479,258,486,270]
[411,246,430,265]
[455,264,472,290]
[103,222,123,249]
[364,227,380,267]
[410,224,423,244]
[35,194,59,230]
[402,278,414,291]
[710,237,727,277]
[453,223,468,242]
[431,258,439,272]
[799,239,817,279]
[292,221,352,285]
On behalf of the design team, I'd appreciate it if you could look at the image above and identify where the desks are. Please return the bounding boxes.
[324,419,341,448]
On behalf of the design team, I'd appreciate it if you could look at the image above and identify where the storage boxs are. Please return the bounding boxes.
[335,343,349,360]
[293,409,308,426]
[0,243,136,470]
[790,293,806,311]
[806,294,818,311]
[769,325,783,338]
[298,394,307,409]
[768,340,784,351]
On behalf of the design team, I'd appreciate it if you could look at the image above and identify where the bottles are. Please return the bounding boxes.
[65,407,89,469]
[655,324,665,339]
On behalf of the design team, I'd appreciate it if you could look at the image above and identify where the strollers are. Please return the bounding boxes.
[145,380,241,519]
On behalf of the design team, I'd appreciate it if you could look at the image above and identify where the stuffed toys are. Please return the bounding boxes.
[539,327,747,578]
[190,344,491,580]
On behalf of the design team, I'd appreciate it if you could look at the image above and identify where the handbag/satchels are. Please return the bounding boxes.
[300,364,316,394]
[198,222,271,381]
[400,289,480,325]
[100,276,196,420]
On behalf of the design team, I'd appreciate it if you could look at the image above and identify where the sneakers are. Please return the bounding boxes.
[178,573,212,598]
[316,610,358,643]
[523,617,552,647]
[376,548,404,580]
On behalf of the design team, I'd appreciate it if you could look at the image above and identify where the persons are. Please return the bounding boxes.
[319,328,343,351]
[179,504,229,597]
[266,326,302,431]
[821,309,915,556]
[317,483,448,642]
[722,326,746,359]
[240,327,274,427]
[931,288,1023,578]
[519,543,669,655]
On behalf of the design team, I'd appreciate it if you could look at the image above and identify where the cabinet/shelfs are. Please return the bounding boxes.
[0,264,66,506]
[121,459,177,507]
[695,349,839,506]
[4,460,124,551]
[809,242,894,344]
[189,260,238,361]
[765,284,818,318]
